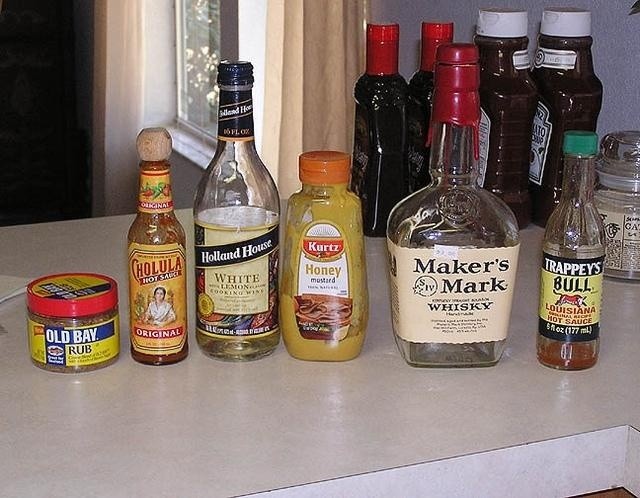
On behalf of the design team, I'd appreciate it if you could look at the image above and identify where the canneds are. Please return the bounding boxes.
[25,273,120,373]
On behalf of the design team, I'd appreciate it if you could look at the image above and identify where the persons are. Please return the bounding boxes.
[137,286,176,327]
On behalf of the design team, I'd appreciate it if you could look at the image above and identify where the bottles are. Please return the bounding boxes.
[342,19,407,238]
[465,9,539,232]
[401,18,456,196]
[190,57,279,359]
[385,40,524,371]
[593,130,639,282]
[122,119,188,368]
[274,146,371,367]
[534,128,612,374]
[529,6,604,225]
